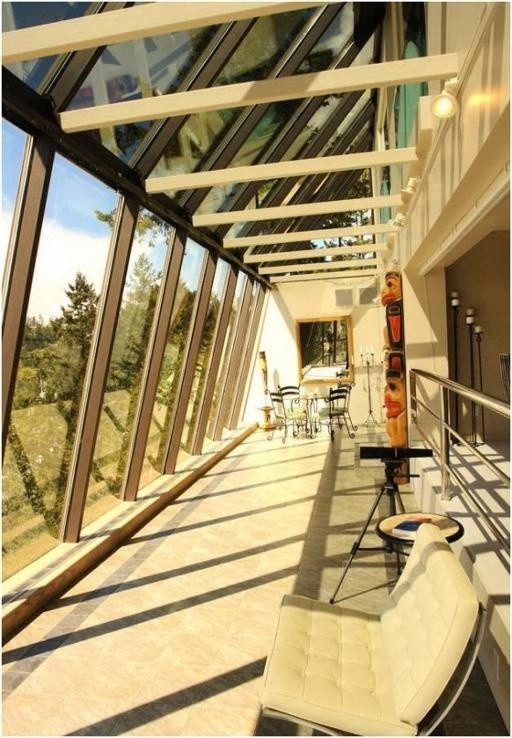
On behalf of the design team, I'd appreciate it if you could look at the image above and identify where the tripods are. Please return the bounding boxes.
[328,459,406,604]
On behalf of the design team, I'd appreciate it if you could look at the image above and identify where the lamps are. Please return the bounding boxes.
[433,78,462,120]
[392,213,406,229]
[401,177,422,195]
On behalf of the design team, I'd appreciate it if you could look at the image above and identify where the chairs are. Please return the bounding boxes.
[266,383,358,443]
[261,523,488,736]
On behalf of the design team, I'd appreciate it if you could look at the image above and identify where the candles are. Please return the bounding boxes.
[466,308,474,315]
[372,344,374,353]
[360,343,363,354]
[366,343,368,353]
[451,292,458,299]
[475,326,481,333]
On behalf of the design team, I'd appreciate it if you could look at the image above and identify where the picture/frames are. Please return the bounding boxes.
[294,315,355,384]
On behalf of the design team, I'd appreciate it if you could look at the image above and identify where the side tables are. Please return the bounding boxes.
[378,512,464,546]
